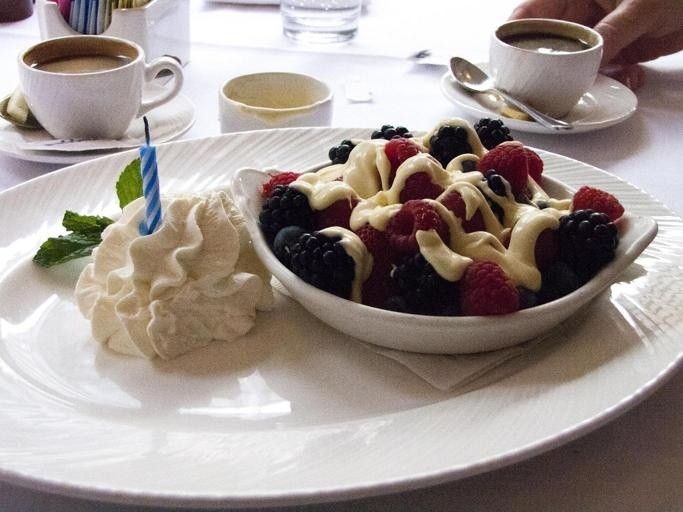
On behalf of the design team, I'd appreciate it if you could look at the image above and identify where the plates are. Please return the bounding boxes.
[440,60,638,135]
[0,81,198,164]
[0,126,683,512]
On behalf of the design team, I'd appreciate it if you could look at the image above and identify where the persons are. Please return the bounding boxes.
[504,0,683,92]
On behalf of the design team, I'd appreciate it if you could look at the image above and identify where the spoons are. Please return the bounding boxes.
[450,56,575,133]
[0,55,181,129]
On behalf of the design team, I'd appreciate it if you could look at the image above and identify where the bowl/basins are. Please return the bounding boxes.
[231,160,658,356]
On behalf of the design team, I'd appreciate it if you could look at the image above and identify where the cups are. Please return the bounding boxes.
[17,35,185,141]
[219,71,334,135]
[280,0,362,50]
[488,18,604,122]
